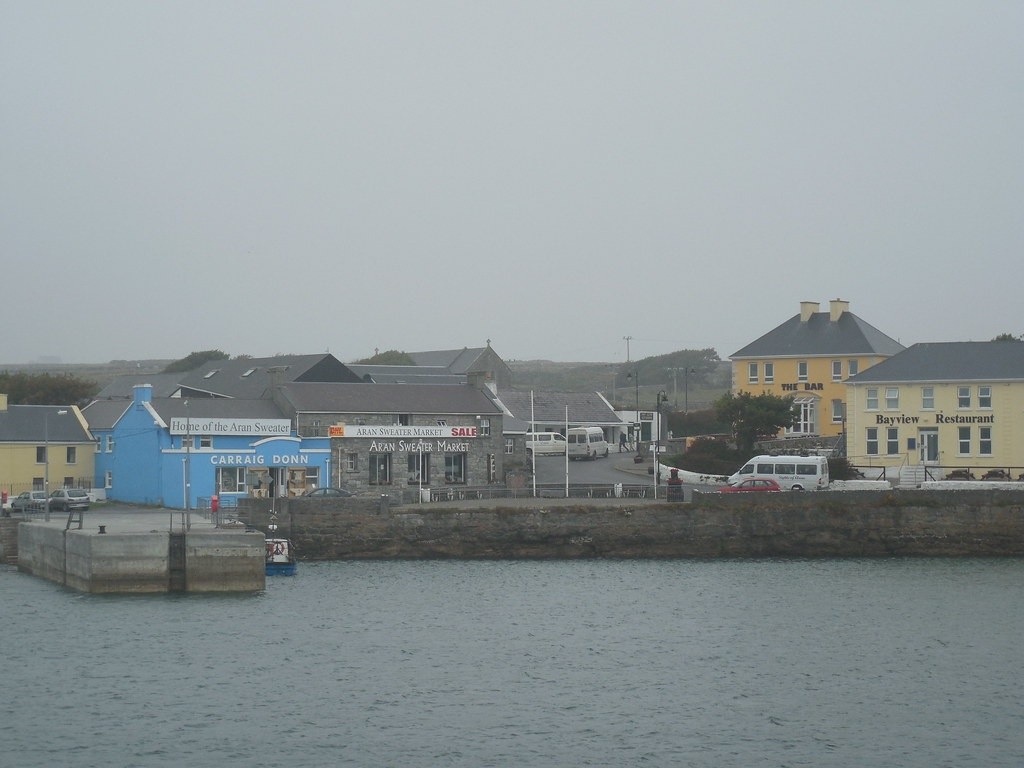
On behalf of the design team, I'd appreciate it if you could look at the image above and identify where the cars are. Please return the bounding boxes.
[303,487,355,498]
[716,476,782,492]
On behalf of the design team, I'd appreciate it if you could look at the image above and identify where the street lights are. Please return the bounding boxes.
[43,410,68,522]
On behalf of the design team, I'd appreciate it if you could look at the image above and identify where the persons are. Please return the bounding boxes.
[618,430,639,452]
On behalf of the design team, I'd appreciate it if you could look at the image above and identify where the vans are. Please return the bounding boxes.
[725,451,830,491]
[568,426,610,461]
[526,431,566,458]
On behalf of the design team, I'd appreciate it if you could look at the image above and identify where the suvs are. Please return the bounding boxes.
[50,488,90,512]
[11,490,54,514]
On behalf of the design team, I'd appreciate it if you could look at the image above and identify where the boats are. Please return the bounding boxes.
[264,467,300,577]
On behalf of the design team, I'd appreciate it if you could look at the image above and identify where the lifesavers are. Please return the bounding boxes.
[270,542,285,556]
